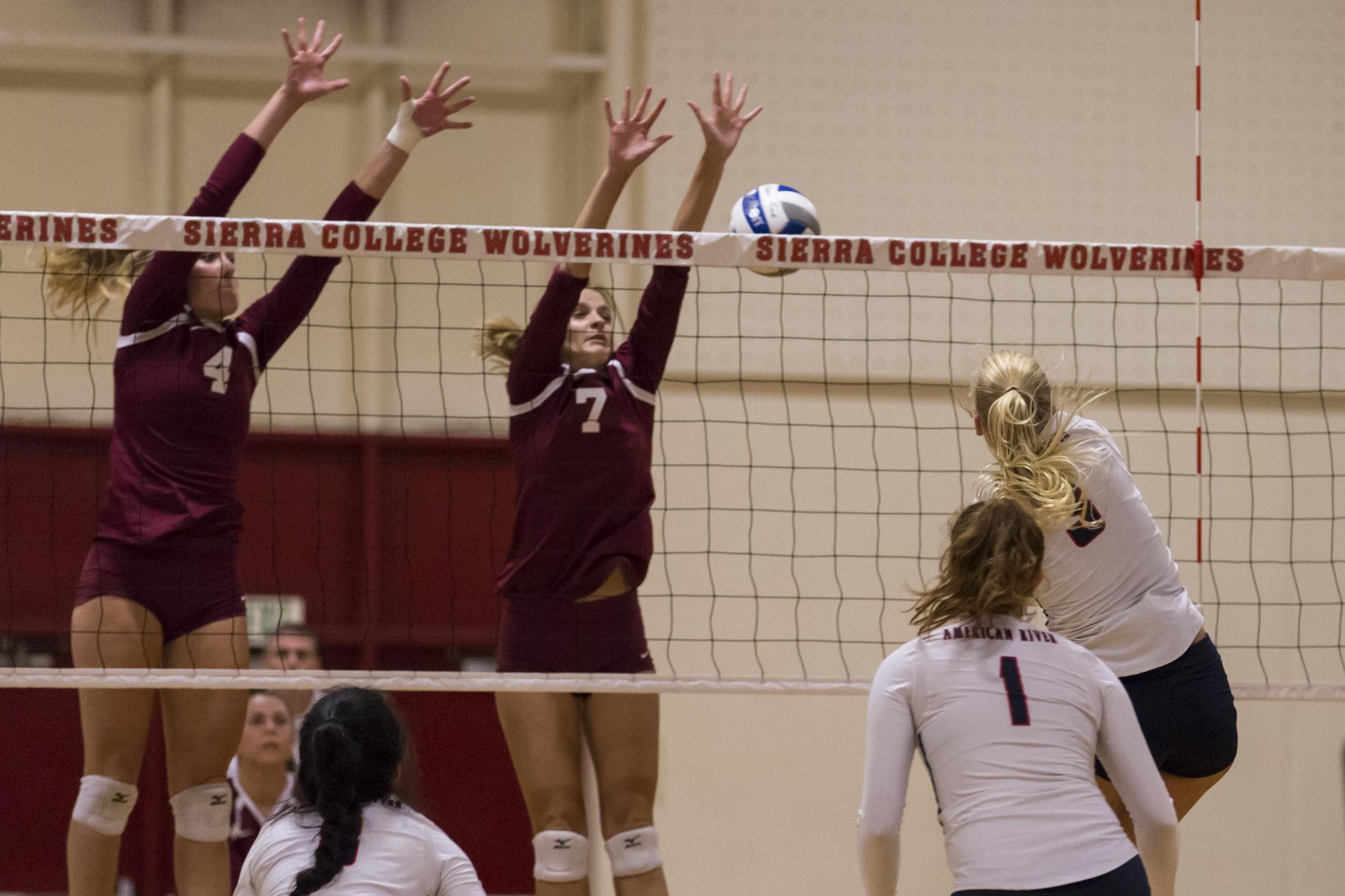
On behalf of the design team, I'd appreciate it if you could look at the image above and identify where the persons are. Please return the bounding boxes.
[226,628,334,896]
[230,685,489,896]
[854,498,1181,896]
[959,349,1239,846]
[477,69,761,896]
[30,17,477,896]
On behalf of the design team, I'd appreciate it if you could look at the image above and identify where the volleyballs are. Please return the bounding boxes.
[725,180,822,279]
